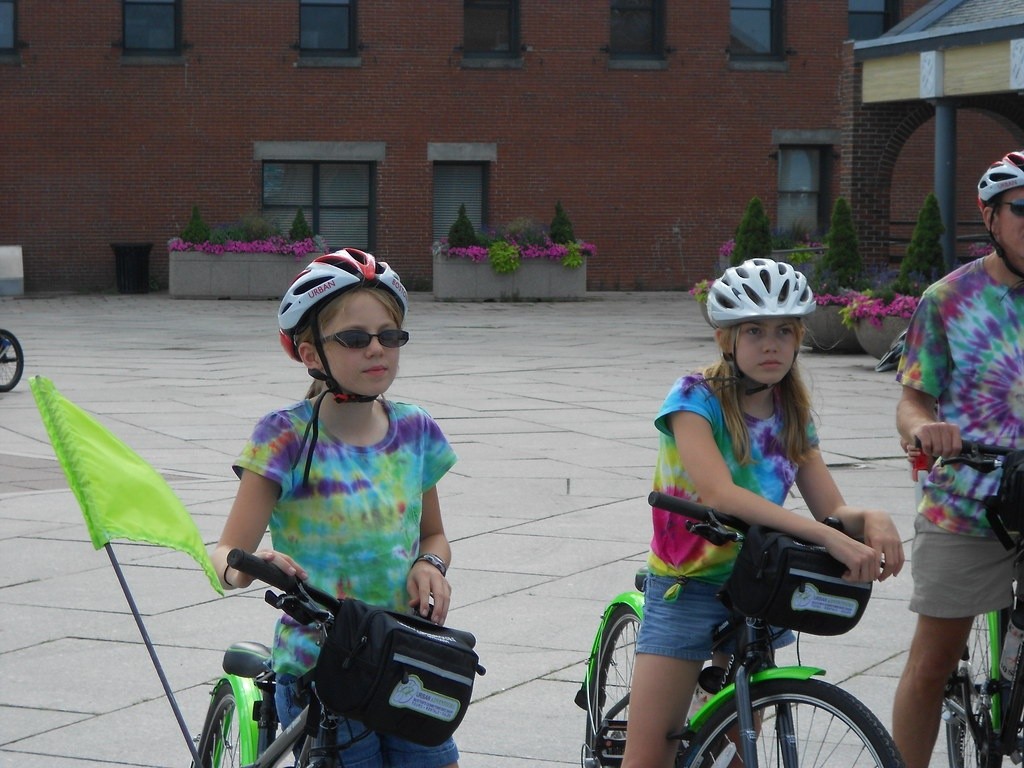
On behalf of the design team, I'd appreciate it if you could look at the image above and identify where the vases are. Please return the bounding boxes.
[432,250,586,302]
[700,303,911,359]
[718,248,829,280]
[169,252,329,301]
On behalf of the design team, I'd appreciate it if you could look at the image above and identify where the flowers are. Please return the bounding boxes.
[167,234,331,263]
[690,239,924,330]
[431,229,596,274]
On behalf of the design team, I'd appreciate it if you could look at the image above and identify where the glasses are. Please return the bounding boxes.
[998,198,1024,214]
[323,329,410,348]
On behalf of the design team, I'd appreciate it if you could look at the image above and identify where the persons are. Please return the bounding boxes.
[621,258,904,768]
[892,151,1024,768]
[208,247,458,767]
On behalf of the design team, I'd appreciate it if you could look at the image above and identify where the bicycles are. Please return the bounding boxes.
[570,493,914,767]
[0,328,25,391]
[190,547,487,767]
[909,439,1024,768]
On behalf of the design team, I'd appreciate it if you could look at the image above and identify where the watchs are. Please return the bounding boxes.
[410,553,446,577]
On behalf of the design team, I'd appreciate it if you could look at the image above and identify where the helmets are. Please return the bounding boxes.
[977,150,1024,201]
[707,257,814,330]
[278,248,407,362]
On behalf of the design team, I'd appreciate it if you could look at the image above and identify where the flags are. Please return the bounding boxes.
[31,375,225,598]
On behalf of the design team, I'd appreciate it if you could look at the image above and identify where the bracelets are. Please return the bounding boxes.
[223,564,233,586]
